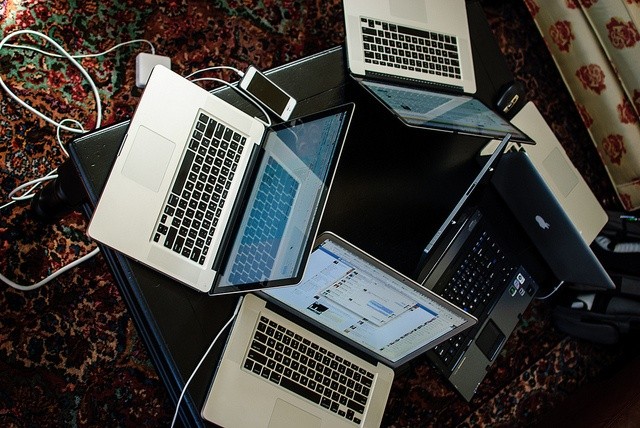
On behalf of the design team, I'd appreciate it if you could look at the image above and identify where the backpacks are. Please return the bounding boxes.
[537,210,640,342]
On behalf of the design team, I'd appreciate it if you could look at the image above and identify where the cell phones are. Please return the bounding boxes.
[237,63,298,124]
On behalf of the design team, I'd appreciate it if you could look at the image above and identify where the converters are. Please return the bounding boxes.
[133,50,172,89]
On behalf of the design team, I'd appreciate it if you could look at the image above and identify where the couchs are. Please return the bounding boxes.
[519,1,637,210]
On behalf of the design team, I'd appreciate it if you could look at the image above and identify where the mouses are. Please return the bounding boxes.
[491,79,524,115]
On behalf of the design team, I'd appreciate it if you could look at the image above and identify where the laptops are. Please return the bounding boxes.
[341,0,538,147]
[412,131,542,405]
[84,63,359,299]
[477,98,619,297]
[198,227,481,427]
